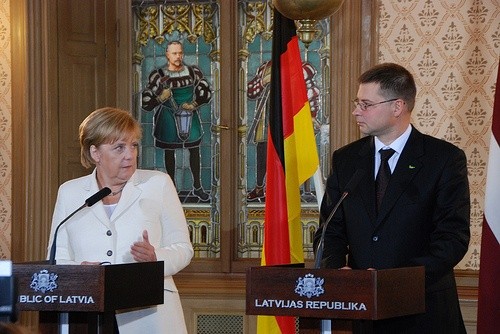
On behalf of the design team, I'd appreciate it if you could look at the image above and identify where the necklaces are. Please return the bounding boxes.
[96,181,126,196]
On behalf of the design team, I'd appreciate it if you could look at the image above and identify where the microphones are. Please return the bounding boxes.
[313,170,360,269]
[48,187,112,265]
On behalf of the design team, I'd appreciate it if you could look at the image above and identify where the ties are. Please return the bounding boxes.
[375,148,396,215]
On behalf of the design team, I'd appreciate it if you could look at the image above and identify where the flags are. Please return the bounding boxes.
[255,6,320,334]
[477,60,500,334]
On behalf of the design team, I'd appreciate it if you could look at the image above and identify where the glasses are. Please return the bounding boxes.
[352,98,398,111]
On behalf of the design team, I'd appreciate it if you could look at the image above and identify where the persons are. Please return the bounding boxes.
[45,108,193,334]
[313,62,471,334]
